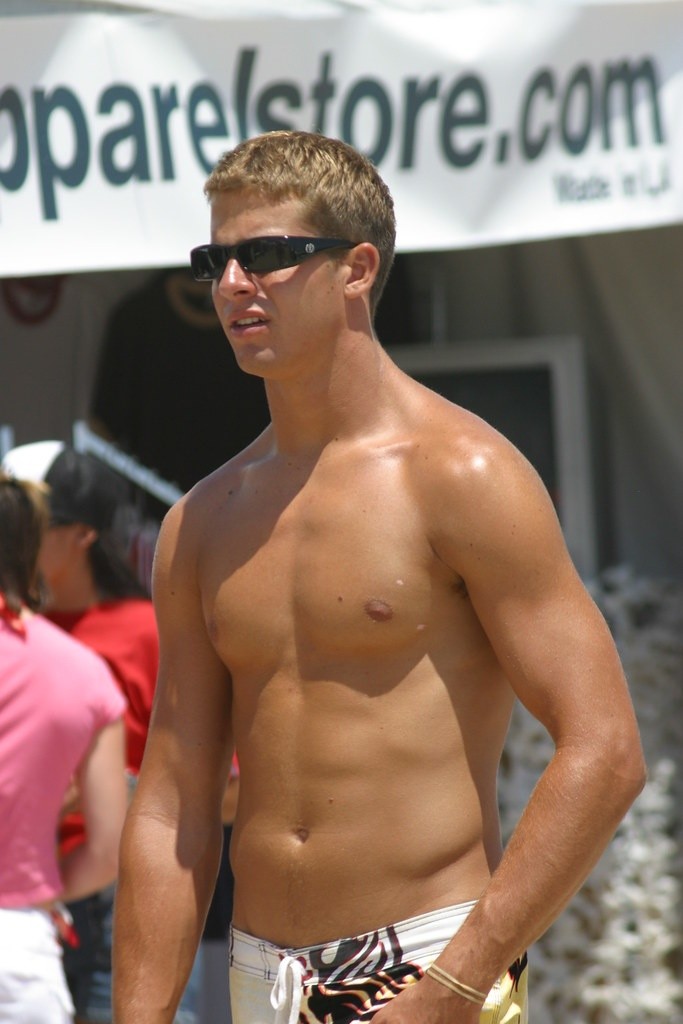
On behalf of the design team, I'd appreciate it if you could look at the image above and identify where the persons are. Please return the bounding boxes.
[0,439,244,1024]
[109,127,647,1024]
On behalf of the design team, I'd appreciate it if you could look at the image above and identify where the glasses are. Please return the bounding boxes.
[191,238,360,281]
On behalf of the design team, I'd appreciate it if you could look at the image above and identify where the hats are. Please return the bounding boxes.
[2,440,123,530]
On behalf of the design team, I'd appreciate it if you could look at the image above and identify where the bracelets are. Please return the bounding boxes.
[426,963,487,1006]
